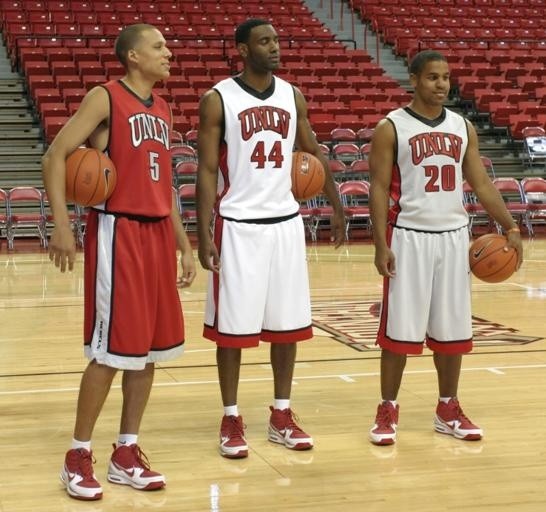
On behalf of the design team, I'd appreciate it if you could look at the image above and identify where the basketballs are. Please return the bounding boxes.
[291,152,325,200]
[66,148,117,207]
[469,233,518,283]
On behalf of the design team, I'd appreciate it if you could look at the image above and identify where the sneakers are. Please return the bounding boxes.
[59,446,103,501]
[265,403,314,450]
[219,412,250,459]
[433,395,484,440]
[369,399,400,446]
[107,441,166,491]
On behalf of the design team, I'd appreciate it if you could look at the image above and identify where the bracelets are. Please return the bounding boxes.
[507,228,520,233]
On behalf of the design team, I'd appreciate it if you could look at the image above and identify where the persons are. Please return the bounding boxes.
[41,23,197,502]
[196,18,346,459]
[367,50,523,445]
